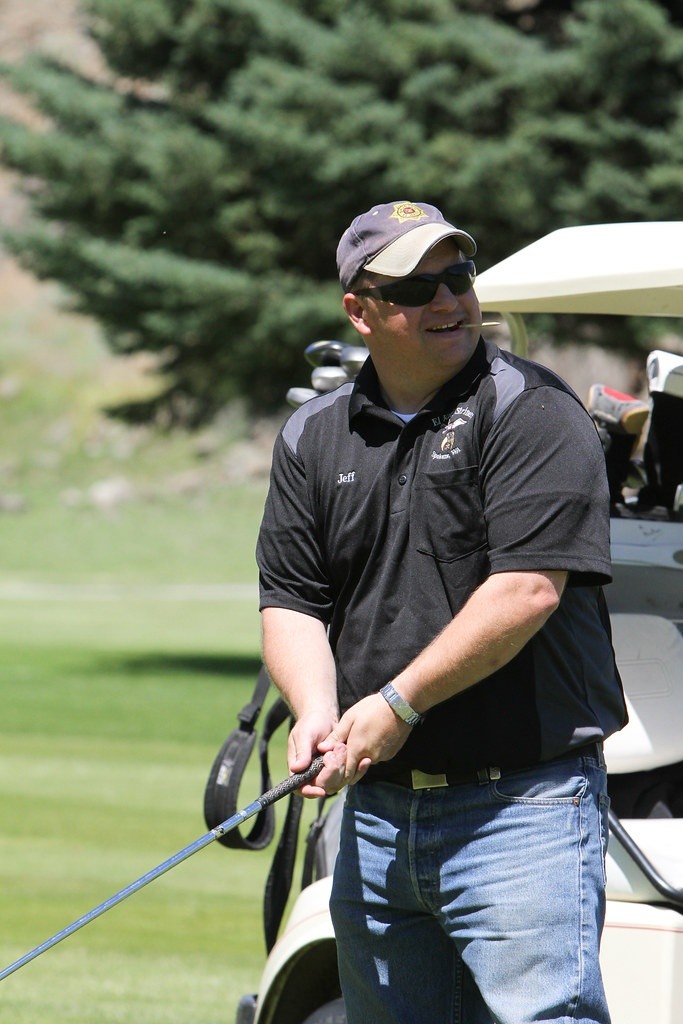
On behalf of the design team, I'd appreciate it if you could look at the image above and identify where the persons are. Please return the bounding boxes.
[256,201,629,1024]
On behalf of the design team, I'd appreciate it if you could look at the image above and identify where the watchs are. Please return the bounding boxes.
[379,682,421,727]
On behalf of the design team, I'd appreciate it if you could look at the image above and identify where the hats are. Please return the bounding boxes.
[337,199,475,294]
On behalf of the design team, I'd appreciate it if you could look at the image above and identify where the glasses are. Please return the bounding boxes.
[352,259,476,308]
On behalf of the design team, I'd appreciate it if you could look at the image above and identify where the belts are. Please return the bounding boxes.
[365,741,604,791]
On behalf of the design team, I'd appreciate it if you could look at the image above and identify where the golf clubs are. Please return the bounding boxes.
[0,756,326,979]
[286,339,372,408]
